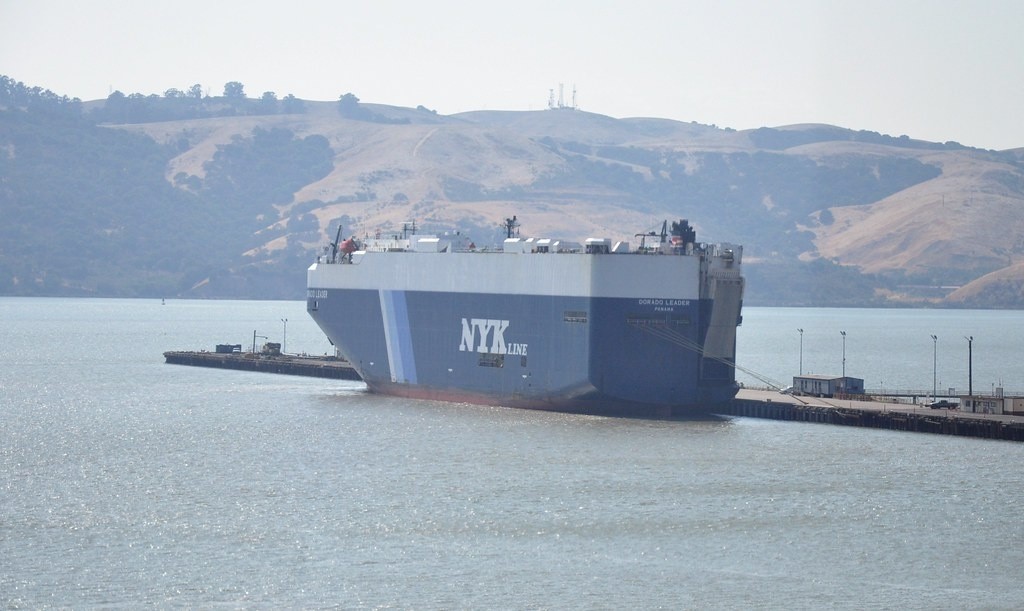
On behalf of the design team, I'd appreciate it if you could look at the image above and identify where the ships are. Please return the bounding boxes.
[304,210,746,411]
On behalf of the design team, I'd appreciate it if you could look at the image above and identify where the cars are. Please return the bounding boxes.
[929,399,959,410]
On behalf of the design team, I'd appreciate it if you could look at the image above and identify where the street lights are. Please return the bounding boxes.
[797,326,804,378]
[839,330,846,377]
[280,317,289,356]
[930,333,938,403]
[964,334,974,395]
